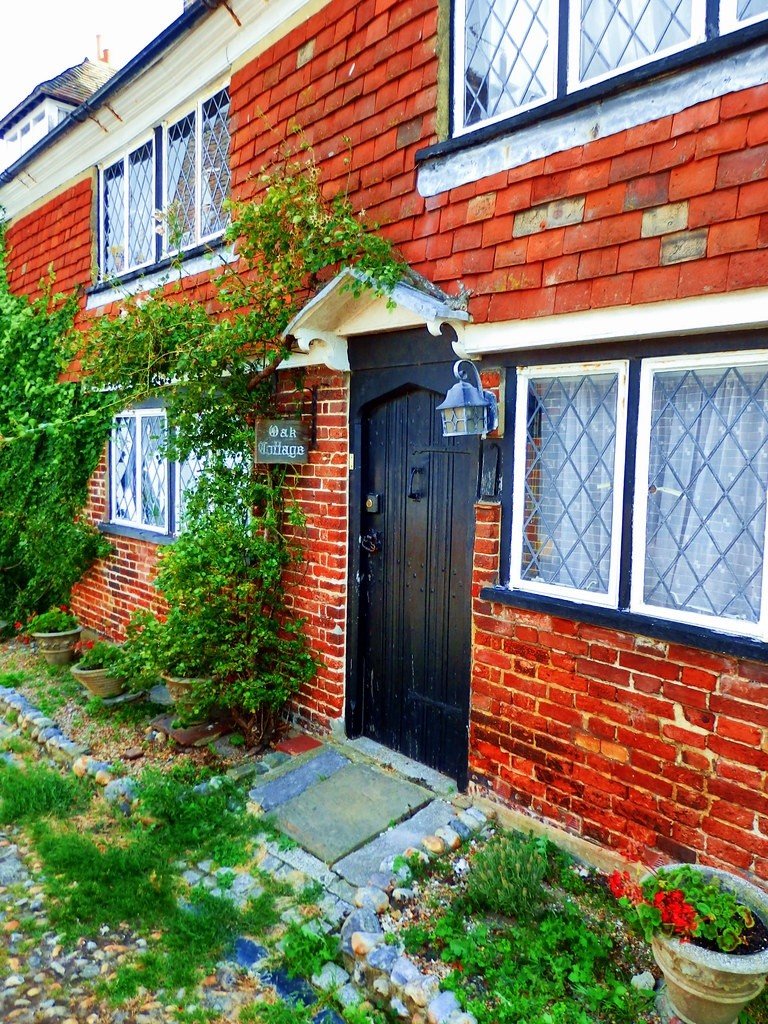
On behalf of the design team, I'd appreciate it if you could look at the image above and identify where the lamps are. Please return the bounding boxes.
[436,357,499,439]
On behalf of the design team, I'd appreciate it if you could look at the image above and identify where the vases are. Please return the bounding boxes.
[70,657,133,699]
[638,863,768,1024]
[31,623,82,667]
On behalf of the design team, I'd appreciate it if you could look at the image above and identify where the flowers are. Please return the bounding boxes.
[607,863,758,954]
[70,637,126,670]
[12,603,81,646]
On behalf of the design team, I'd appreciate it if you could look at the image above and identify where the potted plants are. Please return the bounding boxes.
[104,534,250,727]
